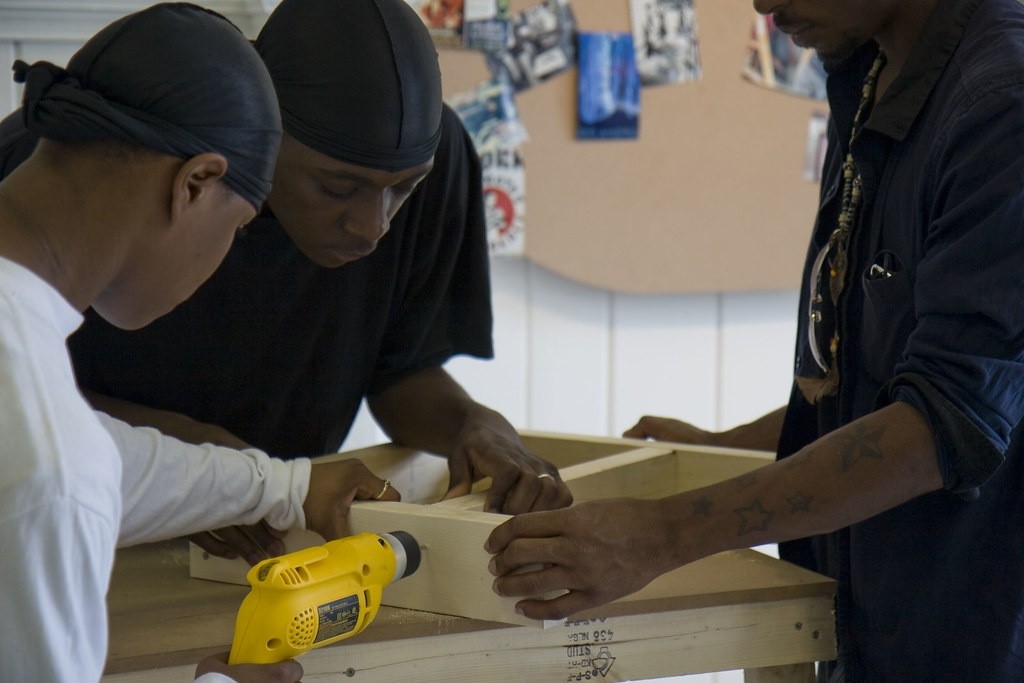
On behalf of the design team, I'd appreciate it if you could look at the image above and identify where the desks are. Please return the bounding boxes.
[101,530,839,683]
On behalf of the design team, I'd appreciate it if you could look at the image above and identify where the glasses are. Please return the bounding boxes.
[808,236,847,374]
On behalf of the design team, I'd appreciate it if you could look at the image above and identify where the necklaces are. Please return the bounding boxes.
[828,45,887,309]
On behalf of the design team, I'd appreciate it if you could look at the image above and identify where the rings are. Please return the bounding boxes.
[375,478,391,500]
[536,472,556,481]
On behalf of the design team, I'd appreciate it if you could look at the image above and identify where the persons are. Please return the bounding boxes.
[0,0,578,683]
[485,0,1024,683]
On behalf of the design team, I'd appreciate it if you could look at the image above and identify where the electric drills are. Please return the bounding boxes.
[229,528,427,663]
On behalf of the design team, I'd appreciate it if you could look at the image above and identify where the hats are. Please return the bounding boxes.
[11,2,283,214]
[254,0,444,172]
